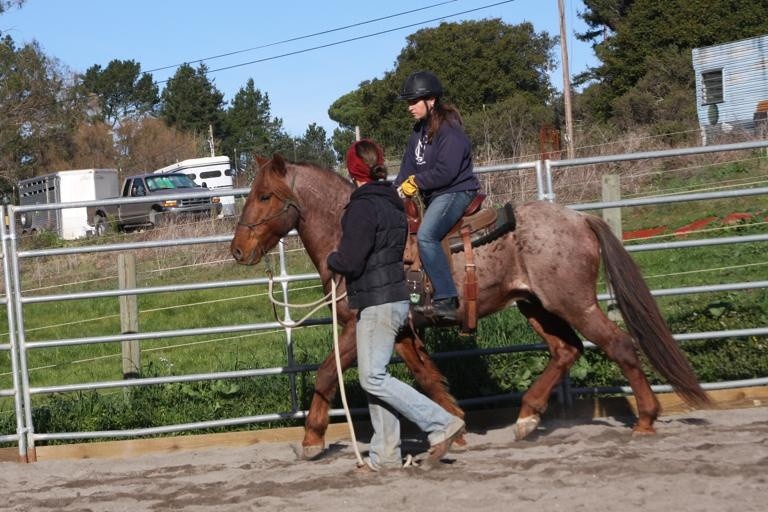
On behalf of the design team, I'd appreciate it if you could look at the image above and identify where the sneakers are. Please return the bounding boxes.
[426,424,468,465]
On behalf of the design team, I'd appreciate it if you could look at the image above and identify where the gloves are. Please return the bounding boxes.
[401,174,418,196]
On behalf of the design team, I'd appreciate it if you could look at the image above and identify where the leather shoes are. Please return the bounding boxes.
[414,296,460,321]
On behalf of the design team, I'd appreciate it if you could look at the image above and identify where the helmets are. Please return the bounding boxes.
[397,73,443,102]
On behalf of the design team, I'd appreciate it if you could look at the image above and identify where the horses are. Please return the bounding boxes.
[228,152,722,461]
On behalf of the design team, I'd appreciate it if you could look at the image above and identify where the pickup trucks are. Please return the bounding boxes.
[85,173,223,238]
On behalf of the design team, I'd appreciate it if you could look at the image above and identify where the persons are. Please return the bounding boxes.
[393,70,484,323]
[321,138,467,481]
